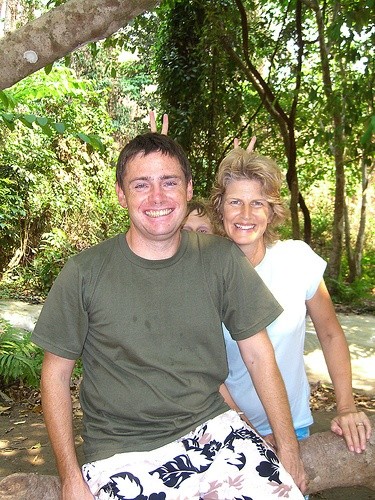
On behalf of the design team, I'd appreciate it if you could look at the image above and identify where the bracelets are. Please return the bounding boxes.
[237,411,244,415]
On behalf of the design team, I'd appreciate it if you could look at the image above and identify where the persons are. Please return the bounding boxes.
[204,149,372,500]
[30,134,308,500]
[149,110,257,239]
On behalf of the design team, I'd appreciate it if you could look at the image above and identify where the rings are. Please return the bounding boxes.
[356,422,364,426]
